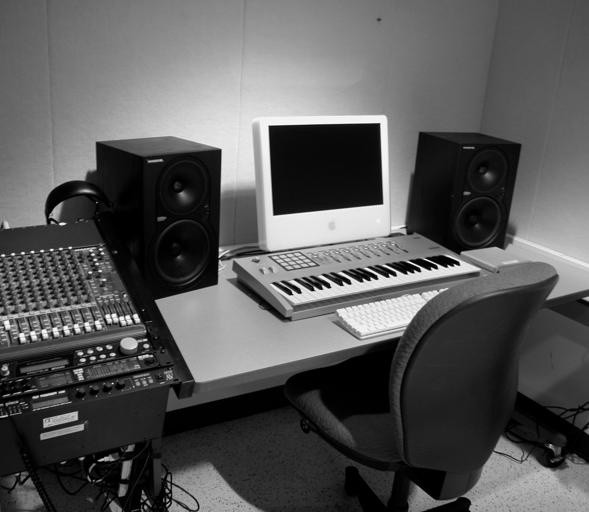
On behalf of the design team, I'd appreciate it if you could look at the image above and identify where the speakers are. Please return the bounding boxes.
[406,132,521,252]
[95,136,220,300]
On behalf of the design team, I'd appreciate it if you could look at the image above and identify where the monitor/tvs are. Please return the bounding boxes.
[251,116,392,252]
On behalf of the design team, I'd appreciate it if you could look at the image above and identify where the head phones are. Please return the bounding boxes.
[46,181,131,267]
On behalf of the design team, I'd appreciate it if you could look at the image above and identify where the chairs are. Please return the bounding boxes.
[281,265,555,512]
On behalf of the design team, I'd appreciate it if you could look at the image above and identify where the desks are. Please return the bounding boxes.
[134,224,589,403]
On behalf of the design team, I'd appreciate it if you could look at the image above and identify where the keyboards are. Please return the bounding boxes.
[337,287,448,340]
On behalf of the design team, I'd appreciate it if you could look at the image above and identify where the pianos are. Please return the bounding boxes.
[232,233,482,323]
